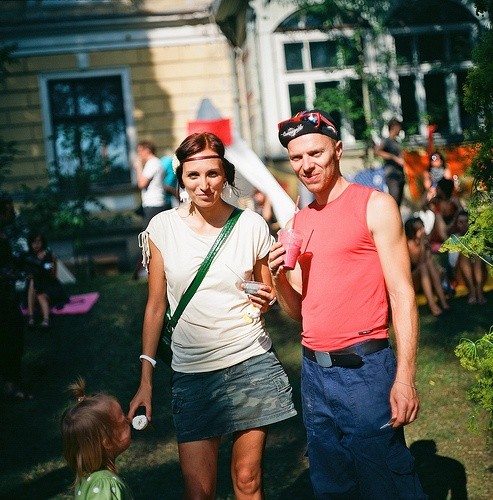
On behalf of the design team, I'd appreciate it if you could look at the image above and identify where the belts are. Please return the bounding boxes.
[303,339,390,369]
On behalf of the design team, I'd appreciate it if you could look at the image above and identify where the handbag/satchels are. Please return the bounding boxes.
[156,309,175,353]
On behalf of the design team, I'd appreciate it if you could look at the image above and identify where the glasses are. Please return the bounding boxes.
[278,111,336,131]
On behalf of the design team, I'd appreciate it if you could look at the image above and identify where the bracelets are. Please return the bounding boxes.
[138,355,157,369]
[394,380,418,391]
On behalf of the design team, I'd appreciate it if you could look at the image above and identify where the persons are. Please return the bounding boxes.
[402,218,453,319]
[267,109,429,500]
[254,182,281,239]
[428,178,458,249]
[451,210,488,305]
[423,151,459,206]
[158,152,186,209]
[375,117,406,208]
[22,231,69,328]
[61,377,133,500]
[137,139,168,230]
[127,132,298,500]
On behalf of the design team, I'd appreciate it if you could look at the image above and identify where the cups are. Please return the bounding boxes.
[277,228,305,270]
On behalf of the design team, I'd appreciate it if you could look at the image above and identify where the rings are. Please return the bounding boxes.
[268,295,276,306]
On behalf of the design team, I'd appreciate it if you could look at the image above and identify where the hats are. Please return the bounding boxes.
[278,109,342,149]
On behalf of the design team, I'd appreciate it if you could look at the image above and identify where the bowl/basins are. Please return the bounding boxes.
[241,281,266,295]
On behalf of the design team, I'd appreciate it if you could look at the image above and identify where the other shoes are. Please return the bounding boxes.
[41,320,49,328]
[28,317,35,328]
